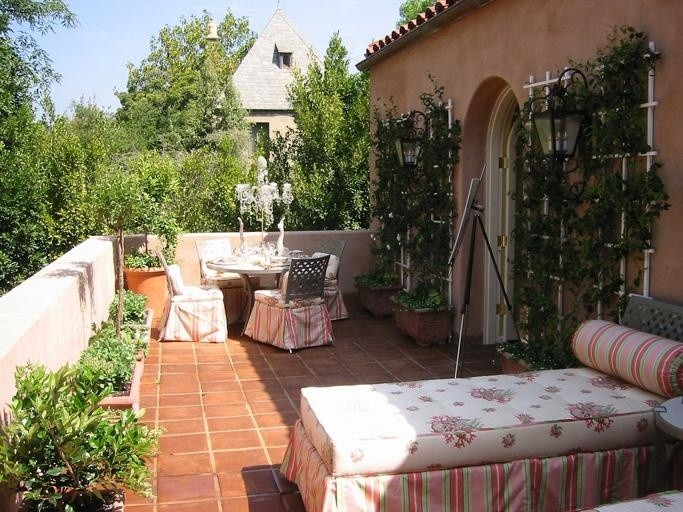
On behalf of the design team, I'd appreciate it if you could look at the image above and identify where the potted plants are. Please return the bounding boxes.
[69,336,145,411]
[115,149,187,339]
[103,287,158,334]
[350,266,405,319]
[389,283,451,345]
[0,356,166,512]
[85,168,156,384]
[488,322,577,379]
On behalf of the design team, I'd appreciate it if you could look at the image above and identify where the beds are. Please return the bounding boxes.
[569,484,682,511]
[279,293,683,512]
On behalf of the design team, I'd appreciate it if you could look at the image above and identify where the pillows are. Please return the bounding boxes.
[565,318,682,400]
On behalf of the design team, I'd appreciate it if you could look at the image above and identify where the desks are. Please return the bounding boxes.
[648,389,683,442]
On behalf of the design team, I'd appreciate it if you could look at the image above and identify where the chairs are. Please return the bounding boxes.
[192,234,259,330]
[278,237,350,323]
[153,242,232,345]
[240,253,338,355]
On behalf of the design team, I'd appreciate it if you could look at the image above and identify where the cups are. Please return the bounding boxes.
[211,240,303,267]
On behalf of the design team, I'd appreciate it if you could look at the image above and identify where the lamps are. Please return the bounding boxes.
[390,109,435,196]
[233,154,294,254]
